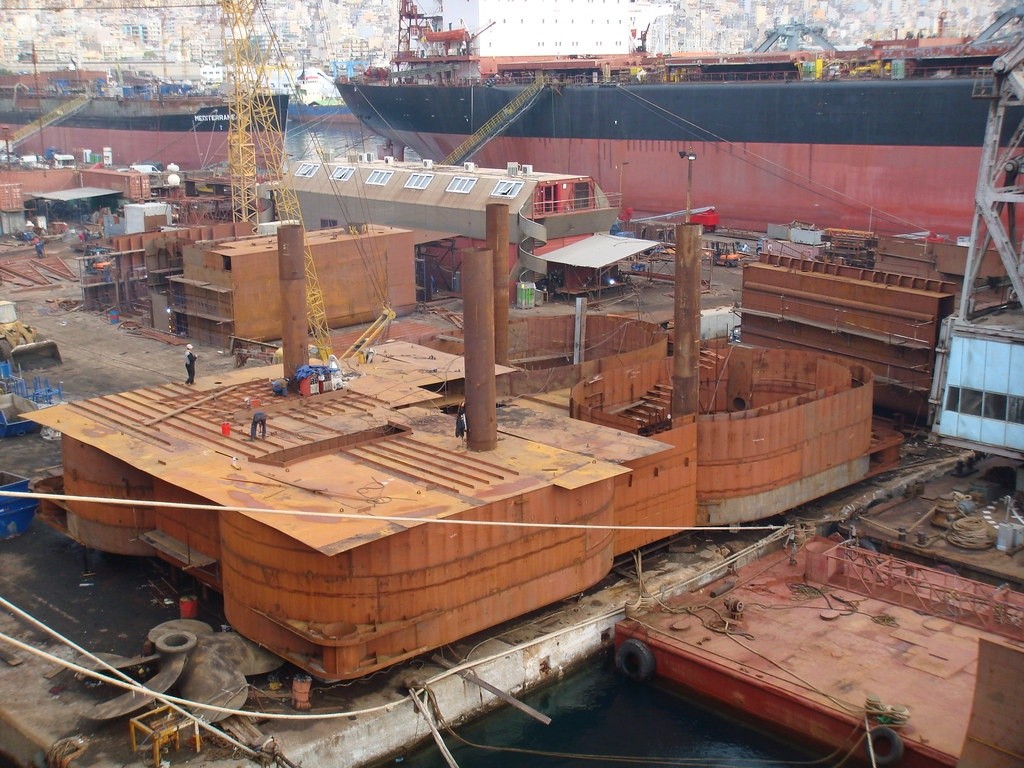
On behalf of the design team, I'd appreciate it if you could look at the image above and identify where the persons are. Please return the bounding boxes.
[79,229,91,242]
[250,413,267,441]
[172,211,179,224]
[272,378,290,397]
[36,241,44,258]
[185,344,198,386]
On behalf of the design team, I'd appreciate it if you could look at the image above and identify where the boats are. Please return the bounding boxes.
[331,0,1023,260]
[0,62,292,176]
[284,67,363,125]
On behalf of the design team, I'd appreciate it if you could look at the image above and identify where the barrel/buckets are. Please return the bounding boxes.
[110,309,119,324]
[290,674,313,712]
[0,361,11,378]
[179,595,198,620]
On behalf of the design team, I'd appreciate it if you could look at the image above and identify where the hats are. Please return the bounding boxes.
[187,344,193,348]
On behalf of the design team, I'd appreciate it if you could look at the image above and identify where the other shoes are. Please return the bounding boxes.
[185,382,196,386]
